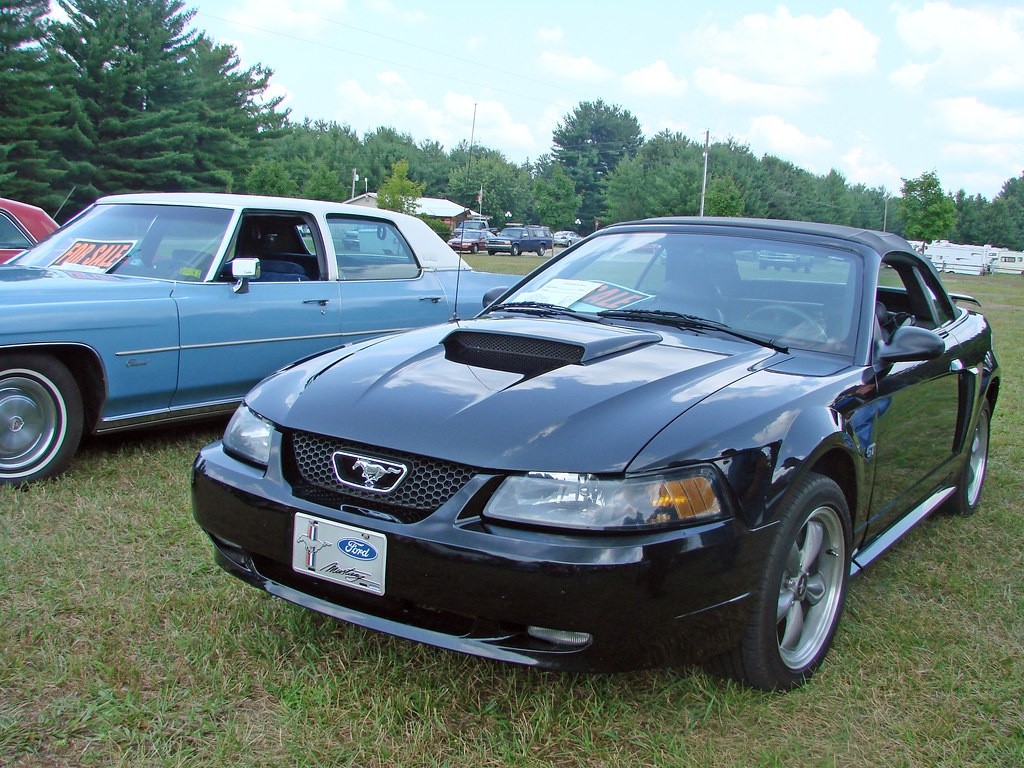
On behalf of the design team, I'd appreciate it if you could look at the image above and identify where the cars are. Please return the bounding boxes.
[0,191,601,493]
[757,247,815,275]
[190,217,1002,695]
[446,229,497,255]
[0,198,63,267]
[550,230,583,248]
[296,223,311,237]
[343,228,362,251]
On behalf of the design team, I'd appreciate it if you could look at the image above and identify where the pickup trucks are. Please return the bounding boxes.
[450,220,499,240]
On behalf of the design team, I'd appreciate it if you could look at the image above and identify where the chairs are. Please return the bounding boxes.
[171,249,312,282]
[826,298,893,344]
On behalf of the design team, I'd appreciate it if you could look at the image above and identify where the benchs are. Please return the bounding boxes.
[265,253,364,282]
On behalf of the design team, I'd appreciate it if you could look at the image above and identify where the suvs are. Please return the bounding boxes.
[485,225,553,256]
